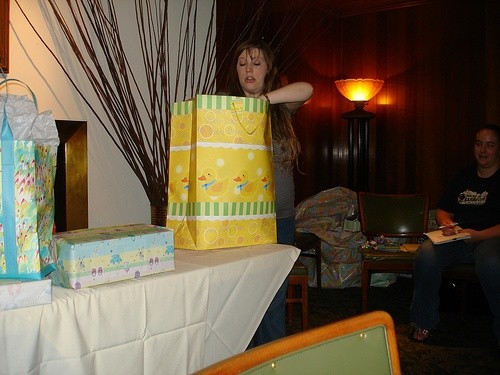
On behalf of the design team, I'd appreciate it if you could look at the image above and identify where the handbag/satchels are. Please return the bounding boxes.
[1,67,58,279]
[164,95,279,249]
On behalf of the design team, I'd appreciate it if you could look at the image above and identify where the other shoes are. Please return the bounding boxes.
[408,327,438,342]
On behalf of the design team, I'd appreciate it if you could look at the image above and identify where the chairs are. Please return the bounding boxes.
[358,191,430,311]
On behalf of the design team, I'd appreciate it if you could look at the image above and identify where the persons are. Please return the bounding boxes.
[409,124,500,342]
[214,38,313,347]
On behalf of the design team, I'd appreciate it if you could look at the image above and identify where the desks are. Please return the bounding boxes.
[0,244,301,375]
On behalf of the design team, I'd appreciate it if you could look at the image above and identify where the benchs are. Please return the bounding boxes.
[193,311,400,375]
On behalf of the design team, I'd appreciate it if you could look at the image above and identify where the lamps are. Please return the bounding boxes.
[333,79,384,191]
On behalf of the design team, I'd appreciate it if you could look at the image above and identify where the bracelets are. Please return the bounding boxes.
[263,92,270,102]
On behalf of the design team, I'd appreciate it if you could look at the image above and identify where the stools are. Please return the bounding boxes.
[285,232,321,330]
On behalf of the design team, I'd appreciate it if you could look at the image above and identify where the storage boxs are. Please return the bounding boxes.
[51,225,174,290]
[0,280,52,310]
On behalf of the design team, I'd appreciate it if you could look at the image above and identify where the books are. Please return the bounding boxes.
[422,226,471,245]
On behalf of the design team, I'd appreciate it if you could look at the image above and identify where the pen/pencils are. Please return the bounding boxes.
[439,223,459,229]
[402,245,409,249]
